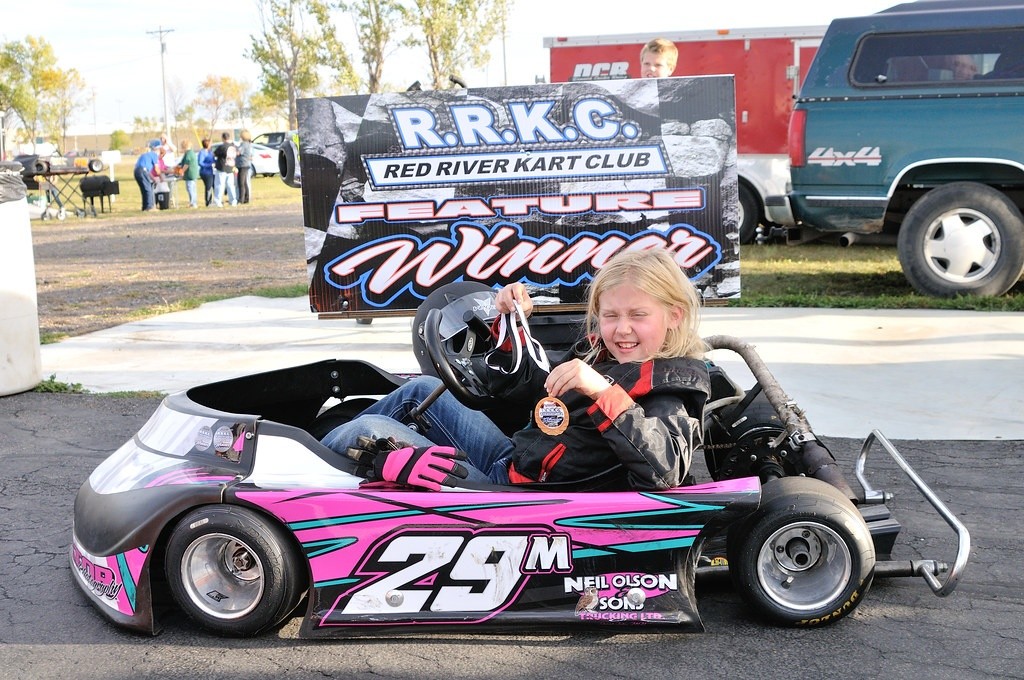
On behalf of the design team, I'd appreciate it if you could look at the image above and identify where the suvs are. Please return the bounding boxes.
[784,1,1024,299]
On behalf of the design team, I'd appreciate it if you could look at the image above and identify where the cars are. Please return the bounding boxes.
[196,142,281,178]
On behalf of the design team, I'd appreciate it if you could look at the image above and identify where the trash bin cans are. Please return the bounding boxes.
[154,182,170,209]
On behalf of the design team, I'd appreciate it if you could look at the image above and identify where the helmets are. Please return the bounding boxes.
[411,281,499,377]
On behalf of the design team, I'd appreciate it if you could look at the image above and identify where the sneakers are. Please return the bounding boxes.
[209,202,224,208]
[186,203,196,209]
[142,206,159,212]
[231,201,238,207]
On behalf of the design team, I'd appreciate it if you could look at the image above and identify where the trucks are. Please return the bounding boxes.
[544,24,986,247]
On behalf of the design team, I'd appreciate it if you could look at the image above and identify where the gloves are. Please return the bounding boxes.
[346,434,470,491]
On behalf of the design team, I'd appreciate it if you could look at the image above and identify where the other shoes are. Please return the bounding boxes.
[239,199,249,204]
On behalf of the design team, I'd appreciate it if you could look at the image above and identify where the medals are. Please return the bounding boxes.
[534,396,571,437]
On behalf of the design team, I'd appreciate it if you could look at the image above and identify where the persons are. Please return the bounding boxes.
[133,133,184,211]
[199,129,253,208]
[45,174,63,208]
[638,37,679,79]
[178,139,198,208]
[321,247,711,489]
